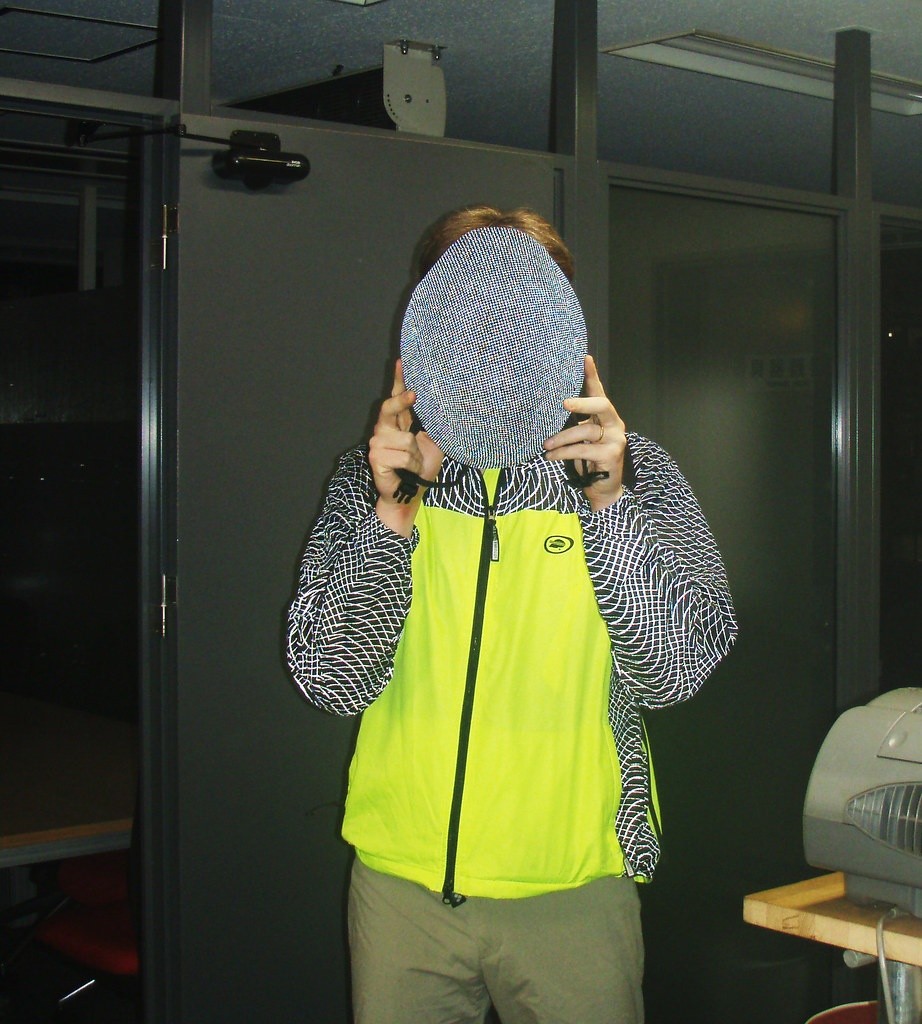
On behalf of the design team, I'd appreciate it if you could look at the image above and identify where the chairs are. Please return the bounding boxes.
[0,850,136,984]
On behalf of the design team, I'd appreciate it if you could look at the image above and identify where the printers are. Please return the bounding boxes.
[802,686,921,920]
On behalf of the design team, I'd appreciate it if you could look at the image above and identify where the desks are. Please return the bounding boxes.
[0,692,141,869]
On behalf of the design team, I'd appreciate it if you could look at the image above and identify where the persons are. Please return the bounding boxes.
[284,205,741,1024]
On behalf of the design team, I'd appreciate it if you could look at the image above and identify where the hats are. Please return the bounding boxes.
[401,224,590,471]
